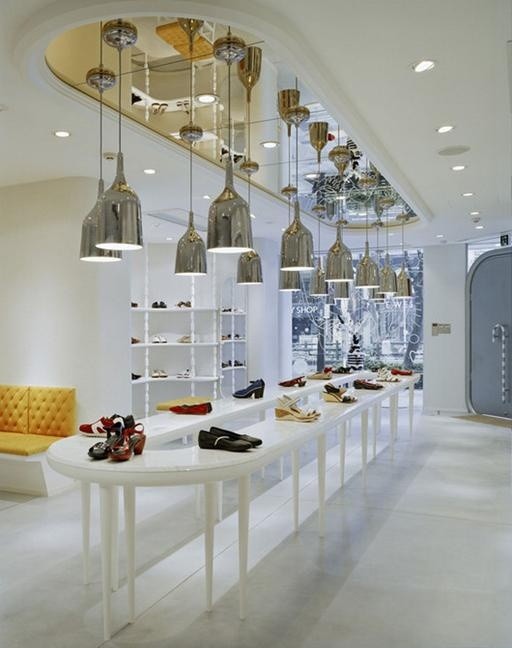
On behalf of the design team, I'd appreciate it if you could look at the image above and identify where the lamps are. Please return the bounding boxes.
[78,18,143,263]
[174,17,262,286]
[277,76,417,300]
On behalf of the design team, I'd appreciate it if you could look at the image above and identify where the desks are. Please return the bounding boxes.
[48,370,422,641]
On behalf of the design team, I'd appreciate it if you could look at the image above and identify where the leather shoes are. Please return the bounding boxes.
[131,302,137,307]
[198,426,262,451]
[308,371,332,380]
[169,402,211,415]
[152,301,167,308]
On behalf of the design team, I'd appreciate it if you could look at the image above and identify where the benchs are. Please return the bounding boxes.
[0,384,79,498]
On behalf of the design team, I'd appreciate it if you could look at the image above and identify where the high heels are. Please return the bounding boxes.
[110,423,145,460]
[278,375,306,387]
[89,423,123,459]
[233,379,265,398]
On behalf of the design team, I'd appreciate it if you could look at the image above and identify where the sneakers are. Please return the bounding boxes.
[223,308,243,312]
[131,368,190,378]
[222,334,239,340]
[332,366,353,374]
[354,378,383,389]
[129,335,190,343]
[177,301,191,307]
[222,360,242,368]
[78,413,133,437]
[376,365,412,382]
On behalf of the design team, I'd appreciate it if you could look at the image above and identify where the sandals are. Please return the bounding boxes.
[274,395,320,422]
[322,383,358,402]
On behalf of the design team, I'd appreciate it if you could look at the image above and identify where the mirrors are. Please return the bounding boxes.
[39,7,423,231]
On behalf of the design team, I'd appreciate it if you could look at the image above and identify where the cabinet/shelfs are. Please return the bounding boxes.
[128,302,247,383]
